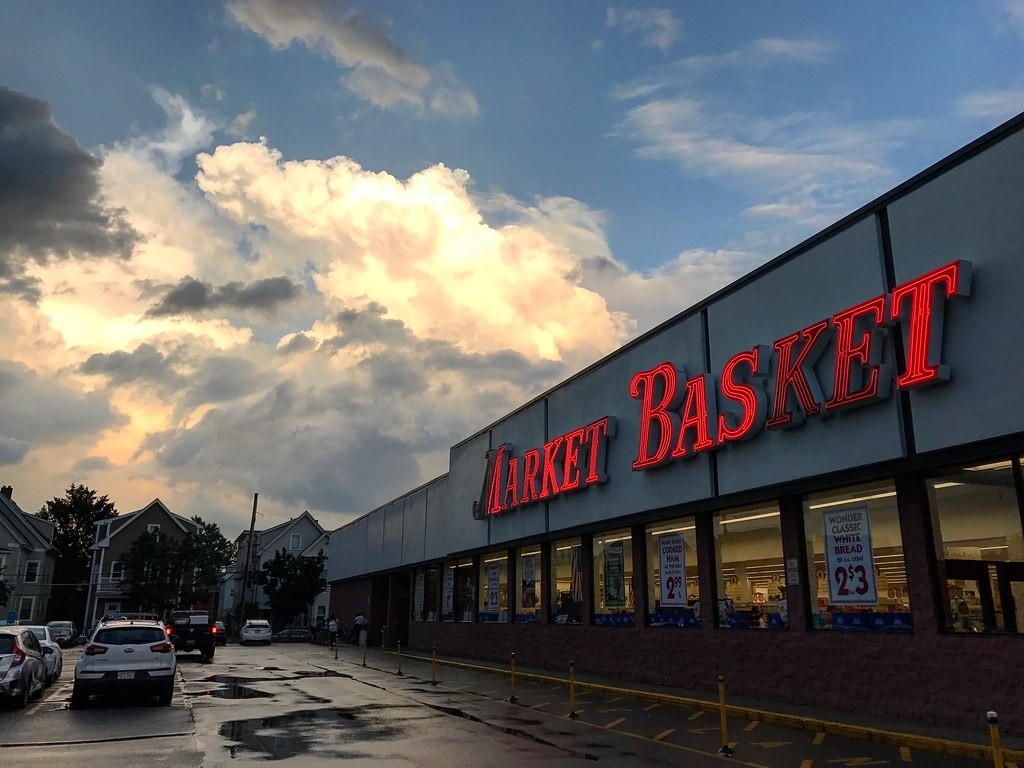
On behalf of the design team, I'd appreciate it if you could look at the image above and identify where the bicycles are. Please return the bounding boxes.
[298,617,354,646]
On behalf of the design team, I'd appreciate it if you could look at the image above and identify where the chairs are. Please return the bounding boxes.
[141,631,153,641]
[104,631,118,642]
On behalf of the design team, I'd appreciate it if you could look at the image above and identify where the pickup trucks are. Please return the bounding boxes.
[160,609,216,659]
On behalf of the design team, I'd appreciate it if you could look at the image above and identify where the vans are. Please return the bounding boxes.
[45,620,78,648]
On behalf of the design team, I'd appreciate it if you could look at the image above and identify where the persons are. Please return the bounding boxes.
[328,614,340,649]
[353,612,366,648]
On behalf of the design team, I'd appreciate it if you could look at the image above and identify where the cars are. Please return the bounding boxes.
[272,629,309,643]
[238,618,272,645]
[16,624,63,687]
[212,621,226,646]
[0,625,54,709]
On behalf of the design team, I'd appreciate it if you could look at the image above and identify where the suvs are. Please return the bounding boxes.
[71,613,179,706]
